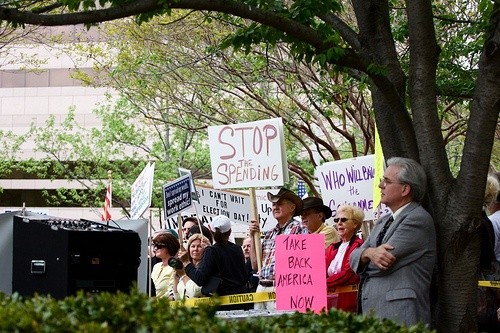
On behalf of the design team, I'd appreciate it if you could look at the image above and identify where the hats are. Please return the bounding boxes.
[301,197,332,219]
[211,215,231,233]
[186,225,212,244]
[267,188,302,216]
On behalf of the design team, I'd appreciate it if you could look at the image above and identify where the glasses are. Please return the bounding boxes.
[334,218,356,222]
[380,179,403,184]
[155,244,165,249]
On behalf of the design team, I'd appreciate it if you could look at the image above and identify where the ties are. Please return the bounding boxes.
[376,216,393,247]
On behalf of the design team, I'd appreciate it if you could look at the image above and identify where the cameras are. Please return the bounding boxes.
[168,257,183,269]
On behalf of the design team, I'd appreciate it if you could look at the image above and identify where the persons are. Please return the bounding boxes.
[298,196,340,255]
[248,188,303,310]
[348,157,438,329]
[476,173,500,271]
[141,204,256,313]
[319,204,366,314]
